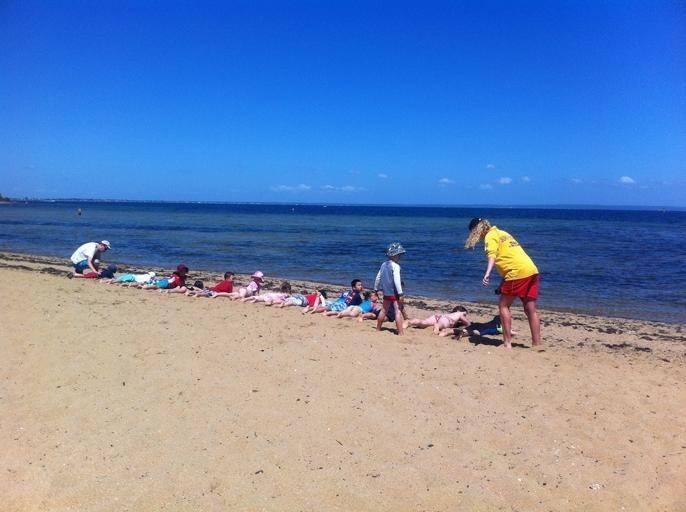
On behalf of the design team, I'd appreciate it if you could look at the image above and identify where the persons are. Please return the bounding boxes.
[68,238,111,275]
[464,217,541,349]
[403,305,503,337]
[371,241,411,337]
[102,266,404,321]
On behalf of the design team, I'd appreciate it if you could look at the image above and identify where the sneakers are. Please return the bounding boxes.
[64,270,75,279]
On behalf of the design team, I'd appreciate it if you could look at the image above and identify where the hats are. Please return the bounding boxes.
[108,264,118,273]
[383,242,407,257]
[98,240,112,250]
[251,270,265,283]
[148,271,156,278]
[173,263,189,273]
[319,290,327,298]
[469,215,488,231]
